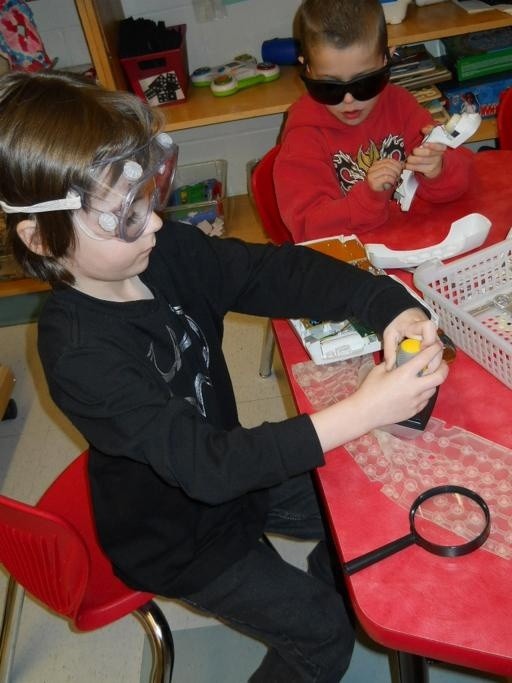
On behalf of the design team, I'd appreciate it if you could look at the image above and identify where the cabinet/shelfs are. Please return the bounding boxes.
[0,0,146,294]
[83,0,512,246]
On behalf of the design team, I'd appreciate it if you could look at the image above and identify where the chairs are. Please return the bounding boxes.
[1,446,173,683]
[245,140,297,380]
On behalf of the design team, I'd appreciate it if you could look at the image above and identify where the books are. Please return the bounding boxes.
[388,42,452,124]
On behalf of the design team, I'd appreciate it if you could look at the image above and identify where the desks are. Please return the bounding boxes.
[257,138,512,683]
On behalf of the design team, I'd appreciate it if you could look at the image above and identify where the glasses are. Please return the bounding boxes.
[300,47,392,105]
[0,132,178,243]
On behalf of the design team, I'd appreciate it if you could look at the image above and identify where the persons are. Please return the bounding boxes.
[272,0,475,244]
[0,69,455,683]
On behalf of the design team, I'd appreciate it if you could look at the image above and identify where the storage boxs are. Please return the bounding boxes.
[433,27,512,122]
[120,23,190,107]
[169,156,228,237]
